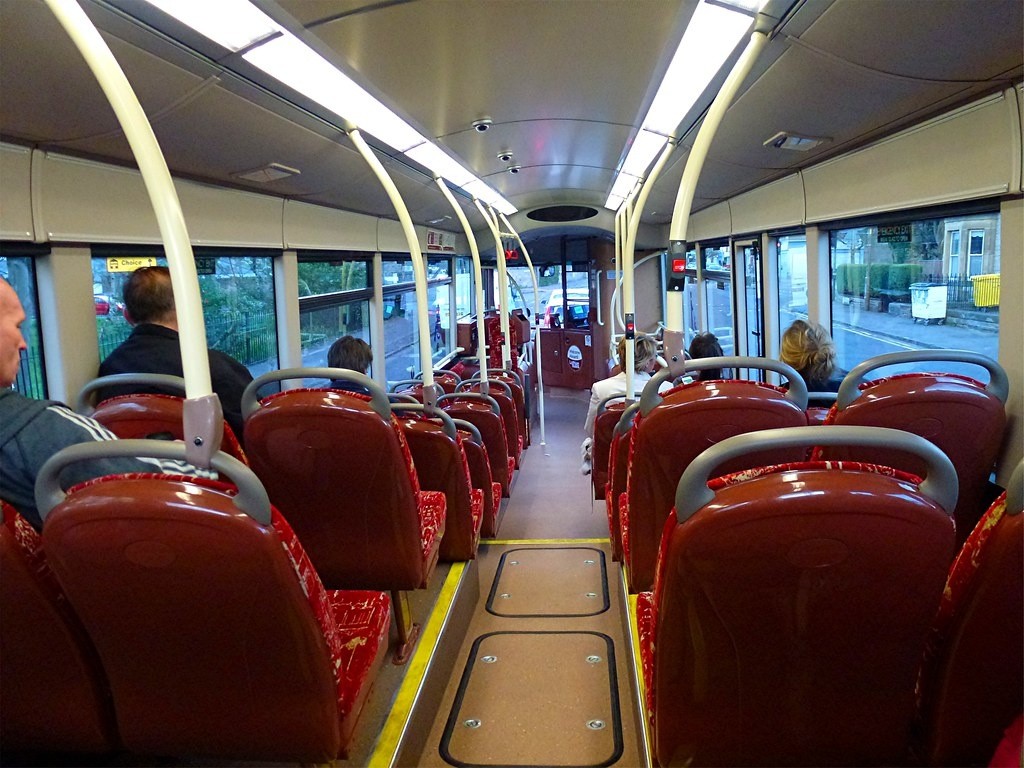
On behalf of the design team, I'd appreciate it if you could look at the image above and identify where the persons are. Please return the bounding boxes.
[95,267,262,452]
[688,332,726,380]
[317,335,403,417]
[0,274,219,533]
[582,332,675,462]
[778,320,844,408]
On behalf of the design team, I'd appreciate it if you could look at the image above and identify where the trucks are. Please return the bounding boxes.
[434,270,520,343]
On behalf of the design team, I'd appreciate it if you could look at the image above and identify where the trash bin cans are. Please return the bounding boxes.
[908,282,948,326]
[970,274,1001,312]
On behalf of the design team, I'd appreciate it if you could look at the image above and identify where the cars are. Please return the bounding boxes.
[93,295,123,315]
[539,286,592,328]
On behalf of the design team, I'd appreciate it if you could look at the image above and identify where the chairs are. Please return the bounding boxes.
[591,349,1024,768]
[0,368,531,768]
